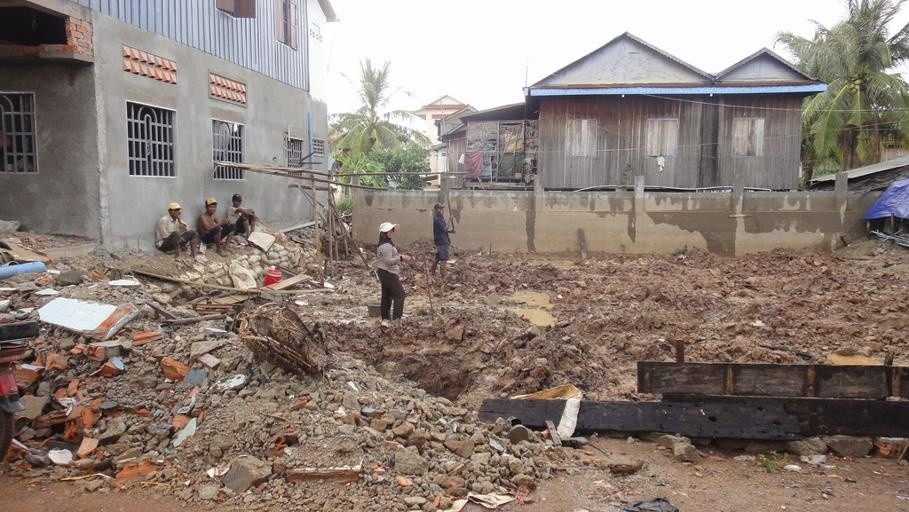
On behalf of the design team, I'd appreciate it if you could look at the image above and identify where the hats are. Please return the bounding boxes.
[232,193,242,202]
[204,196,217,208]
[378,221,401,234]
[434,202,445,209]
[167,201,181,212]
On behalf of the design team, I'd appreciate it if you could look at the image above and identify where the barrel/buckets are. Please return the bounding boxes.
[264,269,282,284]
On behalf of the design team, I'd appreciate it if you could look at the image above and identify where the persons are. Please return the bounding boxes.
[429,202,456,278]
[376,220,413,321]
[197,197,238,257]
[225,194,260,237]
[155,202,199,263]
